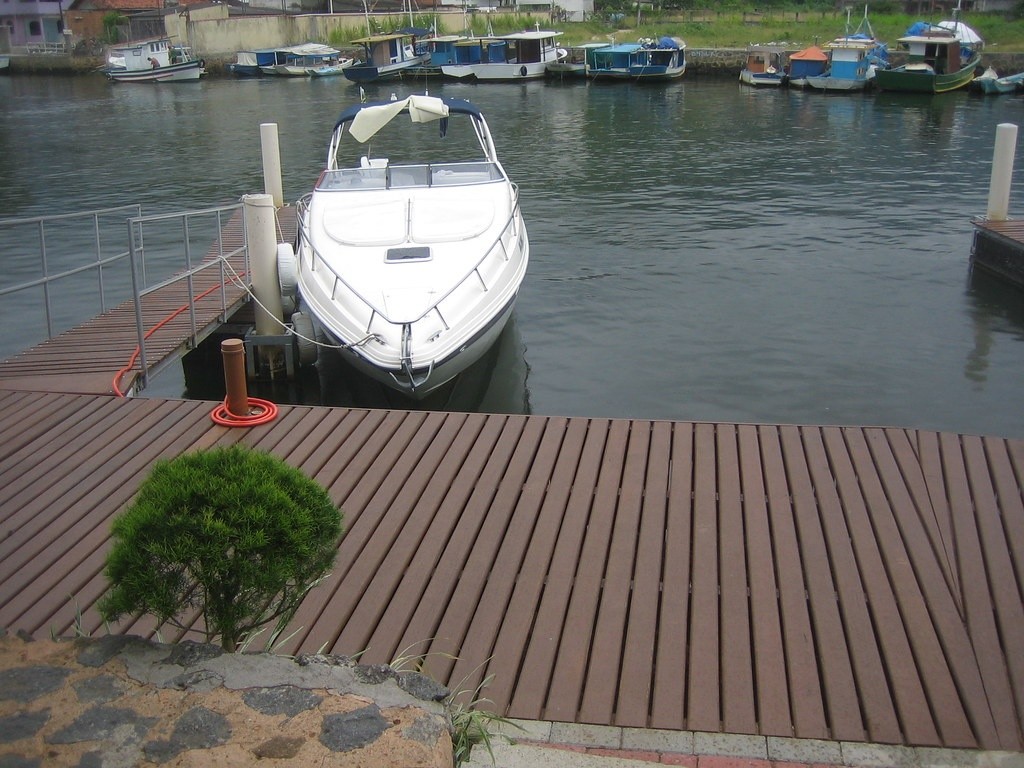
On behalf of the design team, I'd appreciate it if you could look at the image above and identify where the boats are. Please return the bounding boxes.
[878,1,986,96]
[288,96,533,398]
[97,37,207,81]
[228,22,688,85]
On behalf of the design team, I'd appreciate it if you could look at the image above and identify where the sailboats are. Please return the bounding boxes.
[740,4,885,92]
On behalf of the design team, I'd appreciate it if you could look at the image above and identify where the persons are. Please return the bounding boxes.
[147,57,160,68]
[168,46,177,64]
